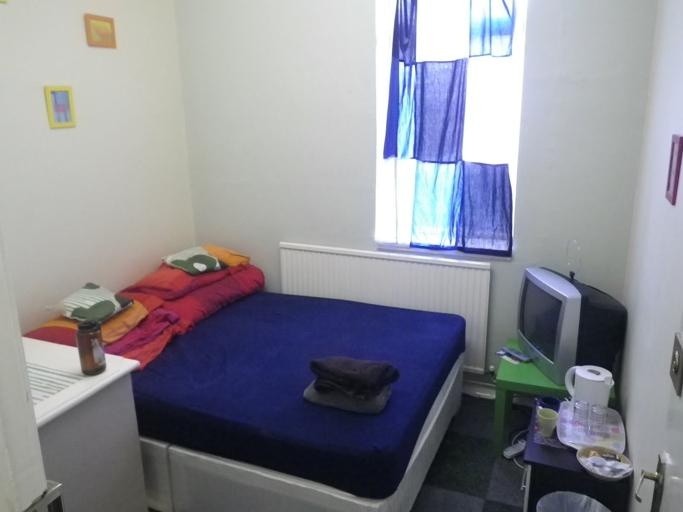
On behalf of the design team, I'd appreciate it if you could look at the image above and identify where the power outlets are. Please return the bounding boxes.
[669,334,682,396]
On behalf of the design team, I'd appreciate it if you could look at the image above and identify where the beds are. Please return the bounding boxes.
[21,244,466,512]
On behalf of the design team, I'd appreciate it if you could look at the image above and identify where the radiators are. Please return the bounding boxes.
[280,241,492,374]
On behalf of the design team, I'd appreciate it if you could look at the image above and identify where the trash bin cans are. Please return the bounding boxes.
[535,491,612,512]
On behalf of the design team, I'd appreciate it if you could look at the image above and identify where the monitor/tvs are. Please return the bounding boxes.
[516,267,627,386]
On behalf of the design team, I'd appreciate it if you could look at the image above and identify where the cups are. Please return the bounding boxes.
[535,405,558,439]
[572,401,606,433]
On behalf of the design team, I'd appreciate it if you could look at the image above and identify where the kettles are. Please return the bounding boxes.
[564,363,615,406]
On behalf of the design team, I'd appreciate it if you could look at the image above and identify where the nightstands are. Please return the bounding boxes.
[20,335,147,512]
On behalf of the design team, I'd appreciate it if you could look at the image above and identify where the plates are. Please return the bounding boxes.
[574,445,634,481]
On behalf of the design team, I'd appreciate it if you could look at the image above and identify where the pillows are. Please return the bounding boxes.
[45,244,228,324]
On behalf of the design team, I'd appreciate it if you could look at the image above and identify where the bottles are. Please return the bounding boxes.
[75,320,106,375]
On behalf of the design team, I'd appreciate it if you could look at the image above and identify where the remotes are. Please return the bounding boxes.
[501,346,532,362]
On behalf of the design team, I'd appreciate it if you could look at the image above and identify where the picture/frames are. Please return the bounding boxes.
[44,84,78,128]
[83,12,118,50]
[663,134,683,204]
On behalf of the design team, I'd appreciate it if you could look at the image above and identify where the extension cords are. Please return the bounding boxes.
[503,440,527,459]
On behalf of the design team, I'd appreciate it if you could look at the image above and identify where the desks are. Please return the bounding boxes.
[495,339,631,512]
